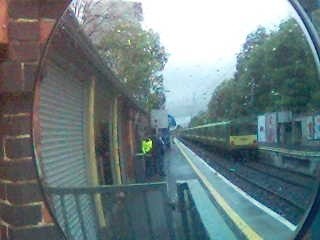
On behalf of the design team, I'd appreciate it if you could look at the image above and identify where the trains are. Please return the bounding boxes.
[179,118,257,150]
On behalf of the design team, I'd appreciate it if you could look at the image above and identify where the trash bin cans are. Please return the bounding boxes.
[135,153,145,182]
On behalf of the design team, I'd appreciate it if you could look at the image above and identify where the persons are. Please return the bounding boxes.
[141,135,168,179]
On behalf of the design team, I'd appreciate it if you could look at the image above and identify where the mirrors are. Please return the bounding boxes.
[30,0,320,240]
